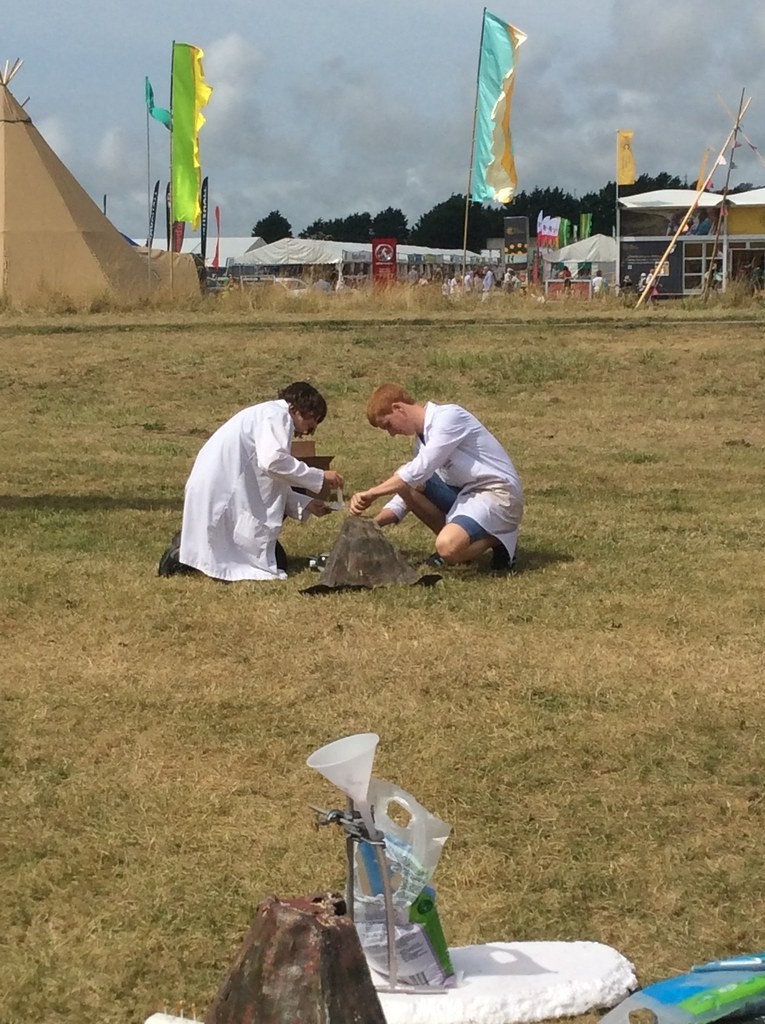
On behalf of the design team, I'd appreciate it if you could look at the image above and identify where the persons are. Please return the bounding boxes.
[347,383,523,571]
[405,264,659,304]
[157,380,343,583]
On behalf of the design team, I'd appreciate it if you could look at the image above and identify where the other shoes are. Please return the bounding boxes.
[423,551,449,569]
[489,541,512,571]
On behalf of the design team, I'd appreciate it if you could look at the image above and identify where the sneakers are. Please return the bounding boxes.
[155,530,191,577]
